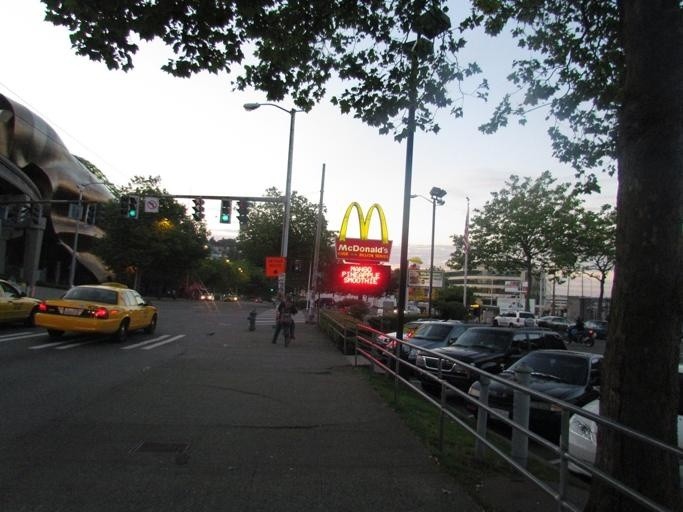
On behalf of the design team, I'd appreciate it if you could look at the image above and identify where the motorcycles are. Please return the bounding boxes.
[280,312,291,347]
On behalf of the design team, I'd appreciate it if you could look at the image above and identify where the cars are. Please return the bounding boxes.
[224,295,240,302]
[0,279,160,342]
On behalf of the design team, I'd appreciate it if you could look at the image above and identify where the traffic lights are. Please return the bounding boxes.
[121,196,139,220]
[237,201,252,231]
[16,206,29,223]
[192,199,204,221]
[220,200,231,223]
[86,203,96,225]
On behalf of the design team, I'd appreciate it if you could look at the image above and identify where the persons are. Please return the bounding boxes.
[271,294,296,344]
[572,316,585,339]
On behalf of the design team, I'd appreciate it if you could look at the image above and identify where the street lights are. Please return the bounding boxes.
[396,8,428,354]
[69,181,114,288]
[243,103,303,299]
[410,187,447,318]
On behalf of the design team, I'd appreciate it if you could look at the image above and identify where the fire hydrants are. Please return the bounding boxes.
[247,309,257,331]
